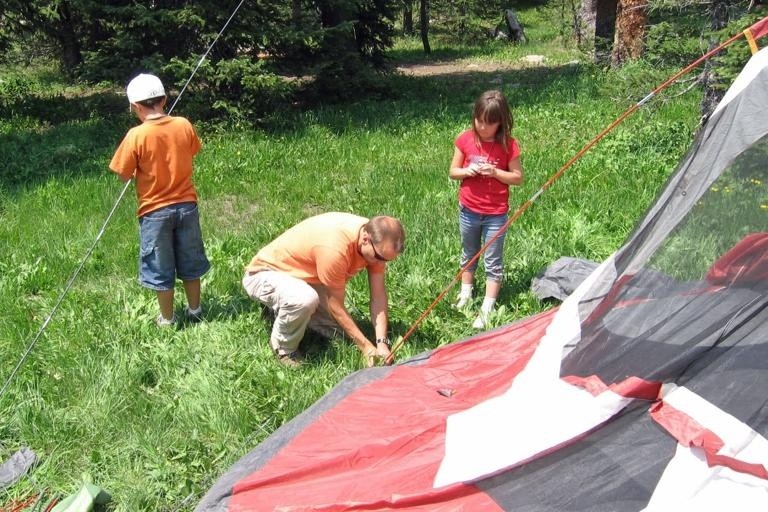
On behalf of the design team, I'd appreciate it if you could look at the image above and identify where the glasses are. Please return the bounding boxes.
[370,239,391,261]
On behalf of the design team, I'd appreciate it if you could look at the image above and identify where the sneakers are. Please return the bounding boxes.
[269,339,305,375]
[473,314,489,330]
[456,293,472,309]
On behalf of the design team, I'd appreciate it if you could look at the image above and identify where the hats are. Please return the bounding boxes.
[127,73,166,113]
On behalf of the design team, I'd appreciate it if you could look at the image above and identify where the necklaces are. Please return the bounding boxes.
[476,135,496,180]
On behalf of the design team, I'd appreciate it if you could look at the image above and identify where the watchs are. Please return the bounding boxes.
[375,338,391,348]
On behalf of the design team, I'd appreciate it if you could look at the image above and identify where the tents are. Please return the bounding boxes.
[193,44,767,512]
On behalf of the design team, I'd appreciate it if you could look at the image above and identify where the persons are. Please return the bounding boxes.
[448,90,521,329]
[109,73,210,326]
[243,211,406,369]
